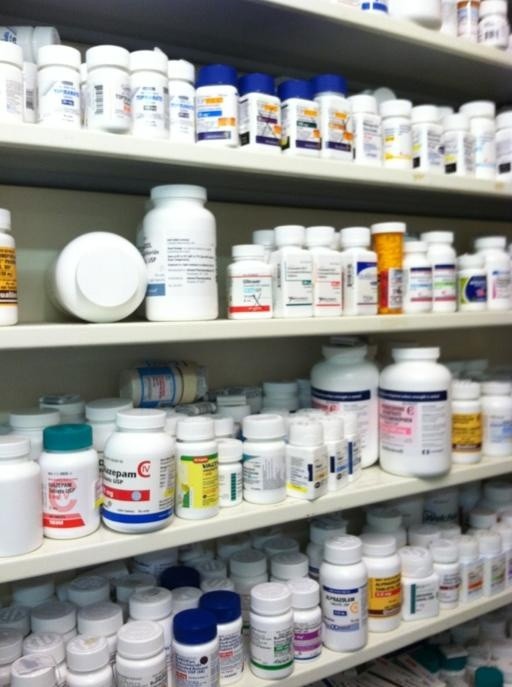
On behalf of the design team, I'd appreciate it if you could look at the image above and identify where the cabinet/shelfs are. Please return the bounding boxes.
[2,2,512,687]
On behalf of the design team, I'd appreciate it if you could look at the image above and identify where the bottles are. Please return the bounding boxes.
[361,0,511,51]
[0,336,512,686]
[43,228,149,322]
[225,222,512,320]
[1,25,62,61]
[142,183,220,321]
[0,40,511,186]
[1,207,22,327]
